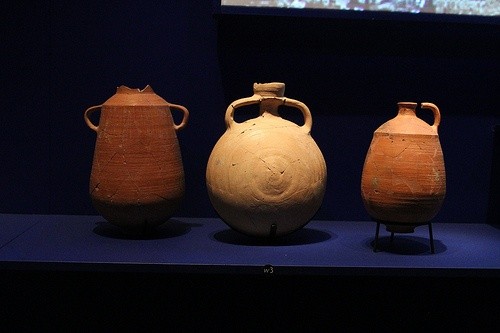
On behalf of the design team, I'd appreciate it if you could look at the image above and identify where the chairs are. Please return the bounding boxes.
[1,210,500,333]
